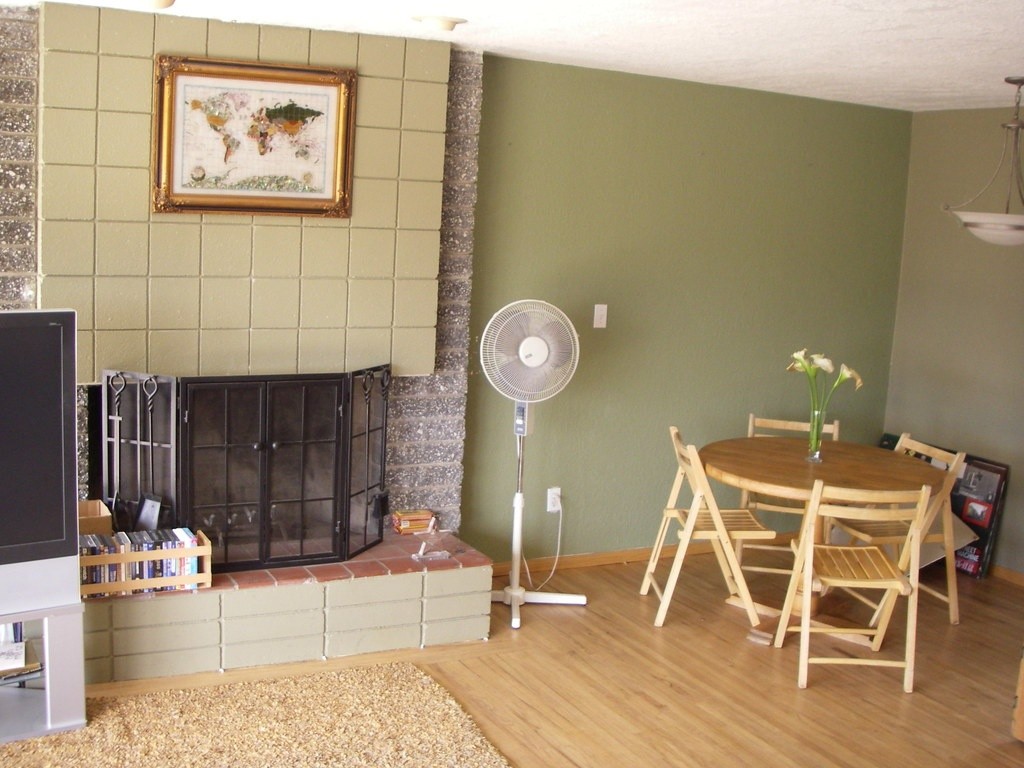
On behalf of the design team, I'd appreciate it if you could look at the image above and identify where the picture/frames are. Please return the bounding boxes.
[152,51,357,217]
[877,434,1009,577]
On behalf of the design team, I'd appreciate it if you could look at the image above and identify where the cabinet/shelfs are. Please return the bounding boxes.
[0,603,87,742]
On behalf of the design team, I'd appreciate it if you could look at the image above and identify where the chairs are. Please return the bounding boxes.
[775,479,931,693]
[734,412,839,575]
[820,432,967,626]
[640,426,760,627]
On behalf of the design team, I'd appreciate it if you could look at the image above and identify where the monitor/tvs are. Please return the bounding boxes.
[0,307,80,616]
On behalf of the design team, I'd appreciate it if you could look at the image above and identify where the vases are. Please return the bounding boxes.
[804,409,829,463]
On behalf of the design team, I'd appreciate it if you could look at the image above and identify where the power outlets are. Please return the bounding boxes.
[547,487,561,511]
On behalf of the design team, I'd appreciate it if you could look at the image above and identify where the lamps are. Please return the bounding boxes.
[941,76,1024,247]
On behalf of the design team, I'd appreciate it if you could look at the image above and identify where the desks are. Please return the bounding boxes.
[695,436,948,646]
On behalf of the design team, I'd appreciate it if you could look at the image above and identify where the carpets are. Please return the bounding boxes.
[0,662,514,768]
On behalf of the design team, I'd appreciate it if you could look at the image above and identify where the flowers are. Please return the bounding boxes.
[785,348,863,449]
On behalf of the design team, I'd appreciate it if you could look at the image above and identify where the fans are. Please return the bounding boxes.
[480,300,587,628]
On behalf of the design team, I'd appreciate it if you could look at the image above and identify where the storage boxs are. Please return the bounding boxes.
[79,500,212,599]
